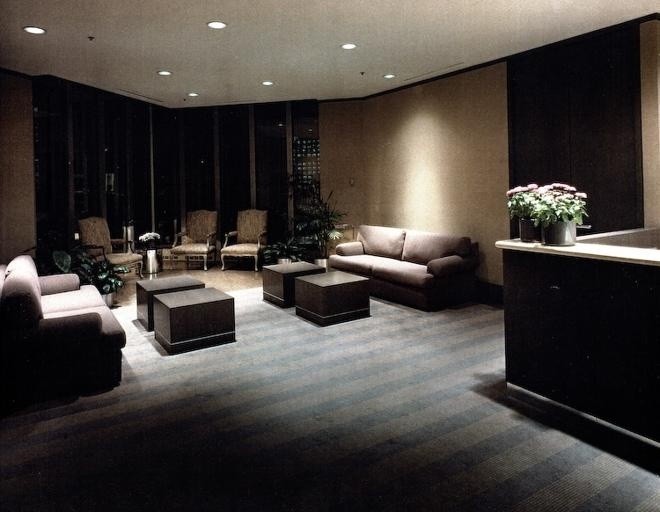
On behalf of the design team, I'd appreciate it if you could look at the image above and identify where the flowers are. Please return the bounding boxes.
[139,232,161,242]
[506,183,590,228]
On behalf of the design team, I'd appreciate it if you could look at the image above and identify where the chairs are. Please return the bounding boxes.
[77,215,145,278]
[220,209,269,272]
[170,210,219,271]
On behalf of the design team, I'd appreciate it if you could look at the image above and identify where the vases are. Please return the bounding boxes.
[144,248,160,274]
[146,239,157,250]
[519,218,577,246]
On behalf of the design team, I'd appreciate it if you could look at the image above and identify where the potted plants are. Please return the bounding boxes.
[288,174,348,272]
[77,251,129,307]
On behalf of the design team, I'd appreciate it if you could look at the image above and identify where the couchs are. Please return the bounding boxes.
[329,225,479,312]
[0,255,126,393]
